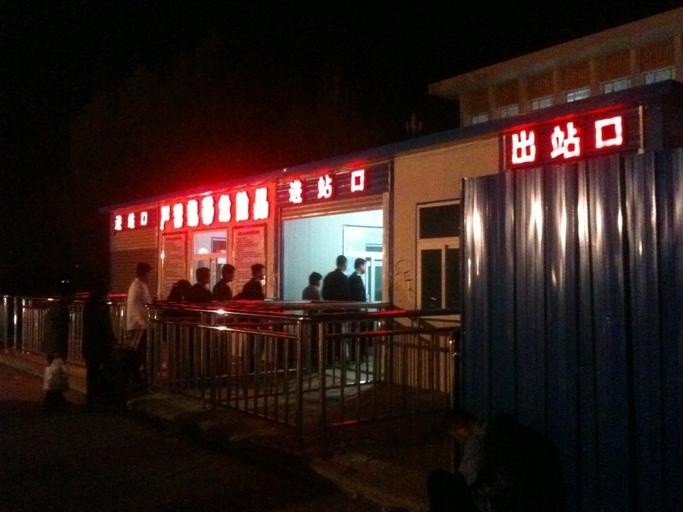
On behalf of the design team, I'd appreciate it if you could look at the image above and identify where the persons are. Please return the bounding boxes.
[163,277,191,379]
[213,264,236,376]
[121,261,156,396]
[425,408,530,512]
[189,266,212,376]
[320,254,351,367]
[301,272,321,366]
[82,271,117,406]
[39,285,77,411]
[346,258,371,367]
[242,263,266,376]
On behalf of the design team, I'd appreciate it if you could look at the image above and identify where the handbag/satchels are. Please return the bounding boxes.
[41,353,73,394]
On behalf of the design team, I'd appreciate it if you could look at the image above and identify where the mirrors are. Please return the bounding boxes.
[341,224,384,312]
